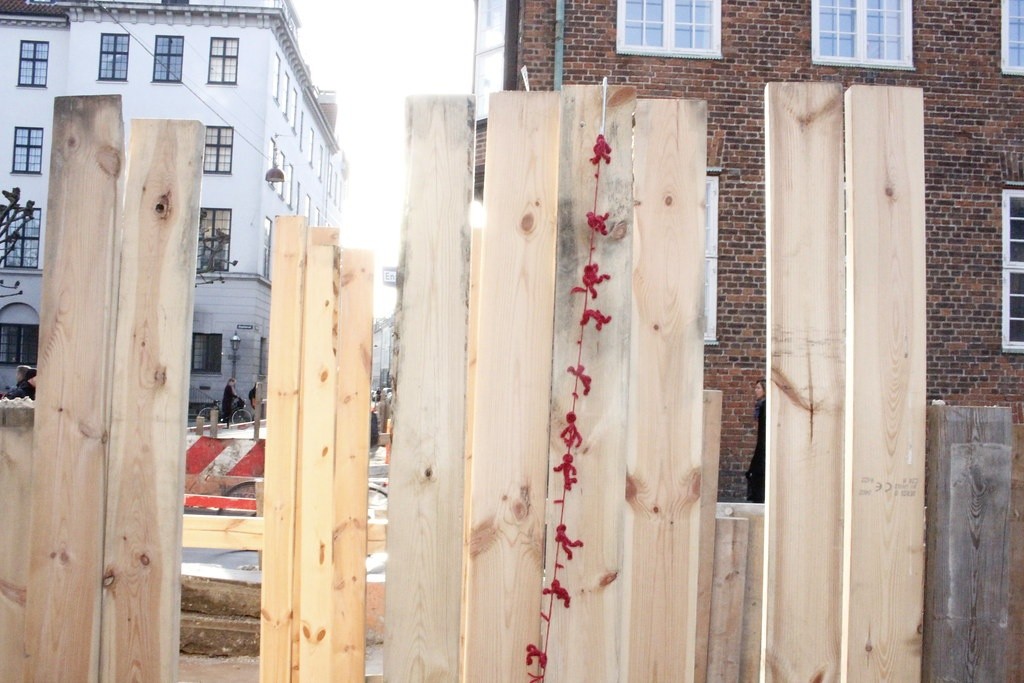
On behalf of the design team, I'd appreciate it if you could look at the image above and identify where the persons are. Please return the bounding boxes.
[222,377,238,423]
[248,383,256,422]
[371,405,379,447]
[3,364,36,401]
[747,379,766,502]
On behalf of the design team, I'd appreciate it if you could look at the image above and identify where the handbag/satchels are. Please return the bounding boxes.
[236,396,246,408]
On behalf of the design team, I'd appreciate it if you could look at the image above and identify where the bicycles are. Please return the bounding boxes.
[198,396,252,425]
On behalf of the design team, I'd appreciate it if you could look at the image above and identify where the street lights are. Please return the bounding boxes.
[229,331,241,389]
